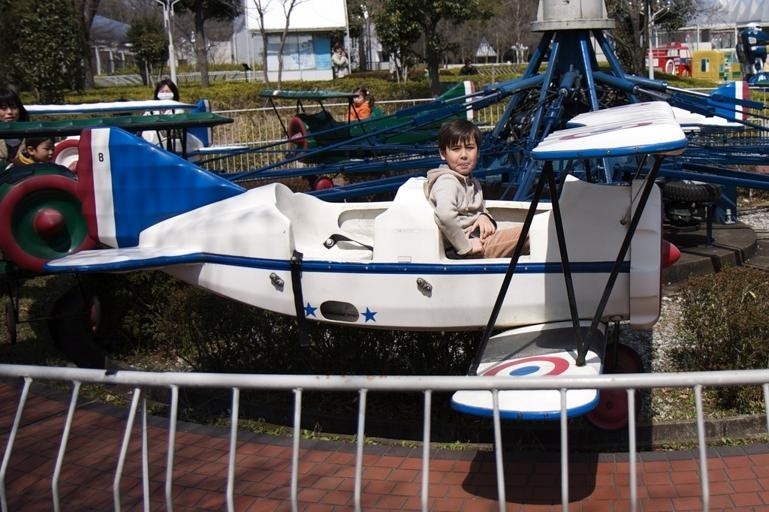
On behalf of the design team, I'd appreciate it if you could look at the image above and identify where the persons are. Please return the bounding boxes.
[344,87,375,122]
[331,46,349,78]
[426,119,530,259]
[142,79,204,162]
[8,136,78,174]
[0,87,67,173]
[458,57,479,75]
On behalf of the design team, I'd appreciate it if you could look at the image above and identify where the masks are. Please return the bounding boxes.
[157,92,173,100]
